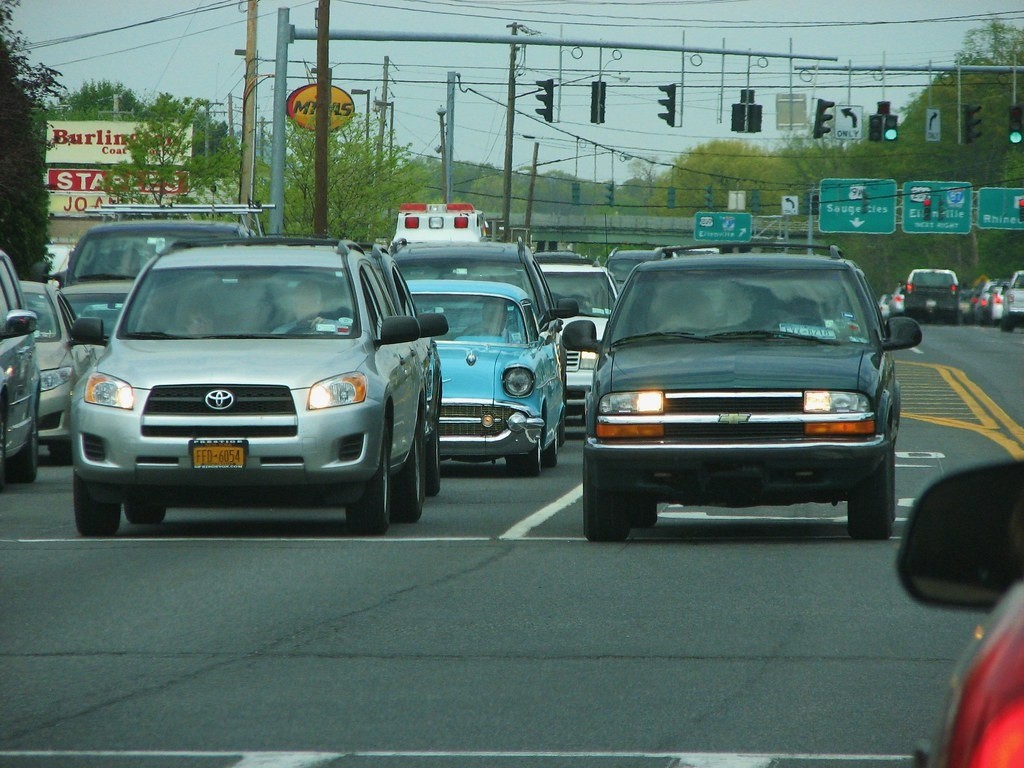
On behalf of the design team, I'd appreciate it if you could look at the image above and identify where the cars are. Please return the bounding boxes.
[0,249,103,483]
[28,218,255,336]
[406,279,566,477]
[387,203,668,401]
[878,268,1024,333]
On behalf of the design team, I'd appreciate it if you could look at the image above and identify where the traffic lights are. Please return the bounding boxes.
[811,194,820,215]
[963,103,1022,144]
[924,199,944,220]
[536,79,554,124]
[1019,199,1024,222]
[812,195,819,215]
[657,83,675,128]
[605,183,614,207]
[813,99,898,142]
[862,192,869,213]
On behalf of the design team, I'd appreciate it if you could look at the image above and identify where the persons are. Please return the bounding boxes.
[271,280,352,336]
[463,300,519,344]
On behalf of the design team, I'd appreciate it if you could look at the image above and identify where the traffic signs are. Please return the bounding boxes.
[781,194,798,216]
[925,108,941,141]
[694,212,752,243]
[836,105,864,140]
[819,178,897,235]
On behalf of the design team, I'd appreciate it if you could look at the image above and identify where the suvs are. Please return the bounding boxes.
[563,241,922,543]
[70,237,449,537]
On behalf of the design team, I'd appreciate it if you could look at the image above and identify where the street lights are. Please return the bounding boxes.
[351,90,394,156]
